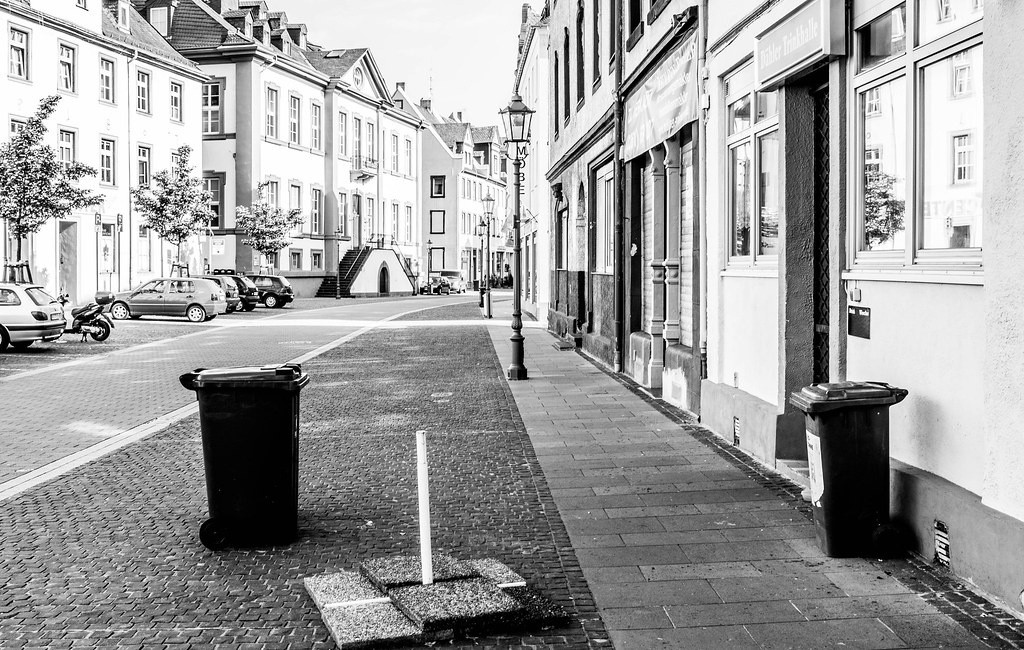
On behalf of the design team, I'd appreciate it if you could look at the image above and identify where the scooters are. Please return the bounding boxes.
[56,287,115,343]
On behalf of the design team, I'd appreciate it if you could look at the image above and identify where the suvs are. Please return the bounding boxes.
[0,282,67,352]
[186,275,240,314]
[240,274,294,308]
[226,275,260,312]
[108,278,227,322]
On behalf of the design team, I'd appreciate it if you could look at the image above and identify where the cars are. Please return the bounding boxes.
[420,277,450,295]
[446,276,466,294]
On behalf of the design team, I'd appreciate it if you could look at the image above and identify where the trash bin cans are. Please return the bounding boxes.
[428,285,433,295]
[178,363,310,552]
[473,279,480,291]
[478,287,491,307]
[788,381,909,558]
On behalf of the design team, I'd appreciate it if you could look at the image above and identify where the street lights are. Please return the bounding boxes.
[427,240,433,295]
[498,84,536,379]
[335,228,341,299]
[480,221,487,307]
[483,194,495,319]
